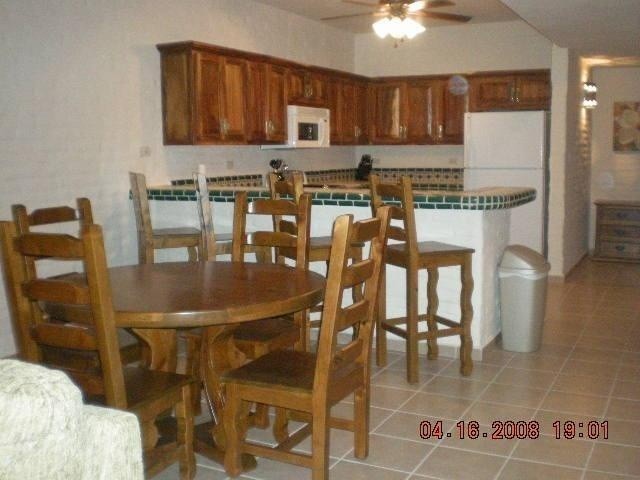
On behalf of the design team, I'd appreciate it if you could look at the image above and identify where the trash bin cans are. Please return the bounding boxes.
[496,245,550,353]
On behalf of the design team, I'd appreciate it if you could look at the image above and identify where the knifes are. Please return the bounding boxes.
[359,153,373,168]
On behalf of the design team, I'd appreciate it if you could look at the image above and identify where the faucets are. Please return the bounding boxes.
[278,163,291,173]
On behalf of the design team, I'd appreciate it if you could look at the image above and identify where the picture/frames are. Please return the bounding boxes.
[611,96,639,152]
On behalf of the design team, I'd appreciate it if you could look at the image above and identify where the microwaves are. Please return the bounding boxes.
[260,106,330,150]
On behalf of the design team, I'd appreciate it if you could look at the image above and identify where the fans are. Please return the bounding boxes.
[320,0,472,23]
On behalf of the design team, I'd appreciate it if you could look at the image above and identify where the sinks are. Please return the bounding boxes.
[303,183,345,188]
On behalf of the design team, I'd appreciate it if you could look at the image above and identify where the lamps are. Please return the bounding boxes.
[580,81,599,110]
[371,2,426,40]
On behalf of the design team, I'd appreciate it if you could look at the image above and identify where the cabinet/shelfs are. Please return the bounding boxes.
[591,199,639,264]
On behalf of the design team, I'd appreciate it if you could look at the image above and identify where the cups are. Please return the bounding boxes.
[274,181,288,194]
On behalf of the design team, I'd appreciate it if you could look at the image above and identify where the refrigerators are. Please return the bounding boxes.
[461,112,545,259]
